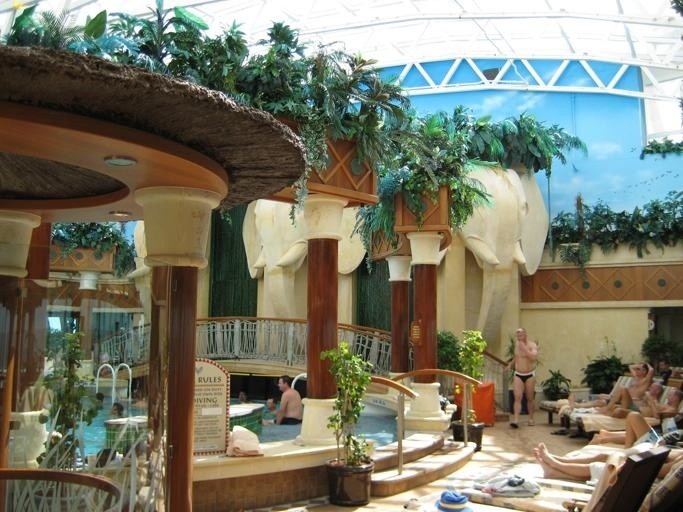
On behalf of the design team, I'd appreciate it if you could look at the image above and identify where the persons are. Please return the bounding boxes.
[265,396,278,416]
[259,374,304,427]
[501,326,538,429]
[107,402,124,416]
[94,392,104,403]
[237,389,254,403]
[532,358,683,482]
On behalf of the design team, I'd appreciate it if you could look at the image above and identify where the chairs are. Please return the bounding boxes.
[539,367,681,442]
[409,438,683,511]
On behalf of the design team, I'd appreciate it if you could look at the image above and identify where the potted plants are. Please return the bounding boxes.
[450,328,485,451]
[320,341,375,507]
[33,328,105,512]
[505,335,543,414]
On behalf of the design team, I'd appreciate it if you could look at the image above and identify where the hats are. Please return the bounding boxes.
[434,489,474,512]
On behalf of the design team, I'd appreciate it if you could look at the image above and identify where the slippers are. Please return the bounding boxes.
[509,418,535,428]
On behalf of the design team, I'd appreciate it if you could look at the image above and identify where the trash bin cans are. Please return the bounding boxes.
[510,390,534,414]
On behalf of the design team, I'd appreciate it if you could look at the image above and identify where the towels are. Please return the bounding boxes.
[226,424,264,457]
